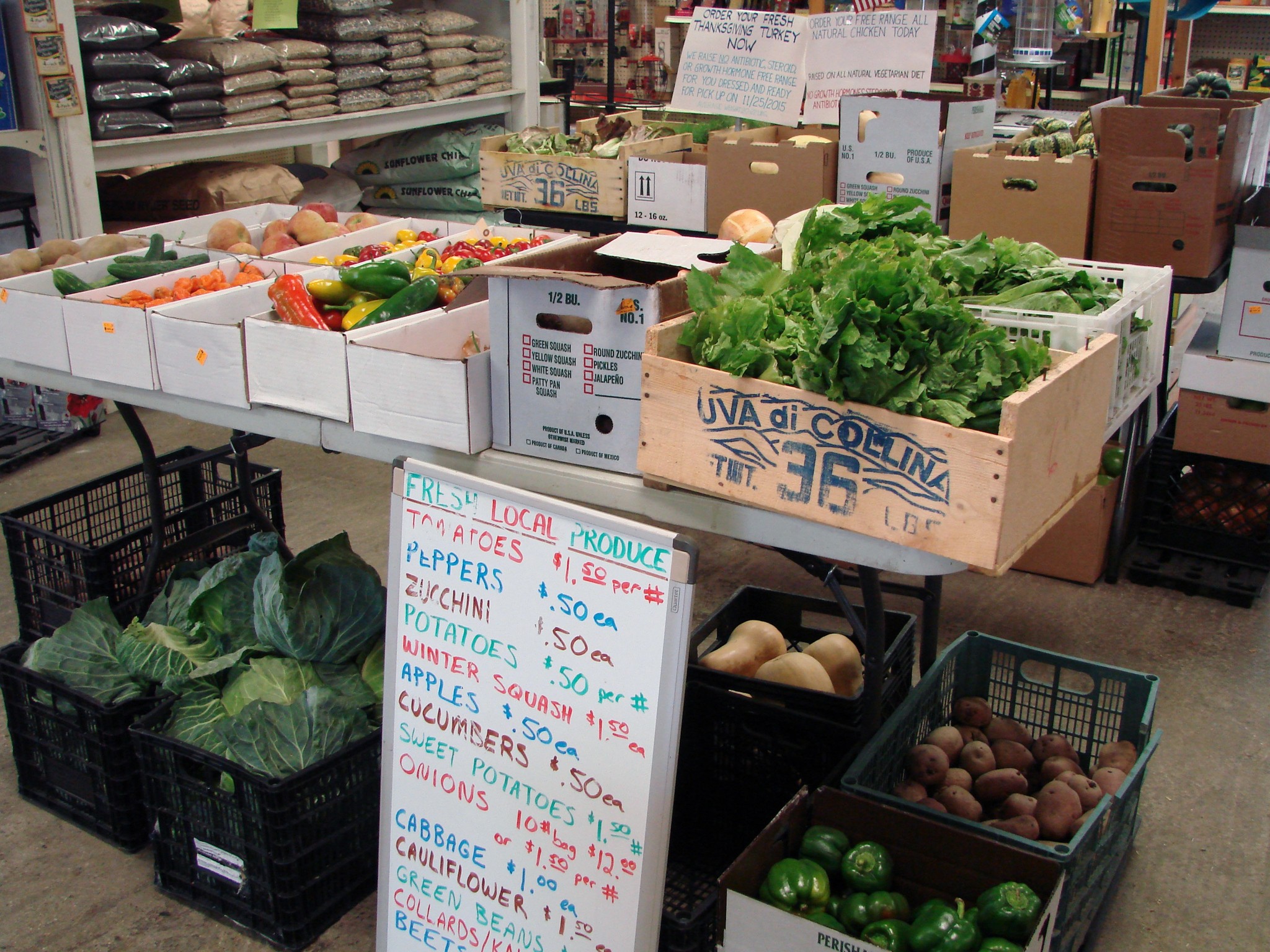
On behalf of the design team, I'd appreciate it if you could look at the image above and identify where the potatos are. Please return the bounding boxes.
[784,697,1139,844]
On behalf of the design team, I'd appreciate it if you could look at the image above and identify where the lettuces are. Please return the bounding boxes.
[683,187,1121,438]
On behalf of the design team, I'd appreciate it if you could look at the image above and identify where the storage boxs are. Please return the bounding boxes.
[703,126,838,236]
[625,151,709,234]
[1014,472,1122,585]
[1085,96,1261,279]
[438,228,782,479]
[1216,223,1268,363]
[638,300,1121,574]
[5,204,580,456]
[1171,313,1270,466]
[835,90,997,230]
[476,127,694,222]
[949,142,1098,263]
[711,784,1068,952]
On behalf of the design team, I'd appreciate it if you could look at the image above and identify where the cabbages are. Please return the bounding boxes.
[13,517,388,811]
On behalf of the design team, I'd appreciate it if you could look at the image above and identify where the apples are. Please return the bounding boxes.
[209,194,375,257]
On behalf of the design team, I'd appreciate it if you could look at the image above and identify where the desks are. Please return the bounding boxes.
[3,359,978,742]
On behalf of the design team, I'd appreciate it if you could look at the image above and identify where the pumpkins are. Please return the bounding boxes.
[697,610,868,695]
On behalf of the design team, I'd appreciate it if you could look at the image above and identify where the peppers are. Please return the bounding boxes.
[753,846,1038,952]
[270,231,554,381]
[97,253,272,315]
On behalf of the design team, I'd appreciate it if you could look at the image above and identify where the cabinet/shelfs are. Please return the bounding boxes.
[0,1,536,419]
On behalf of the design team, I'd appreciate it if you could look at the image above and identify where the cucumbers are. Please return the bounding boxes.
[53,229,209,307]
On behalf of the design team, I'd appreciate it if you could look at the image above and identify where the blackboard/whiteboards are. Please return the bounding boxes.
[376,458,701,952]
